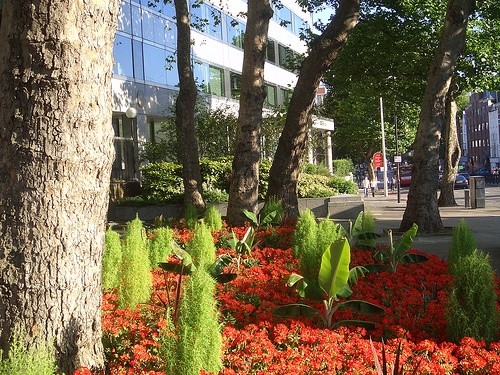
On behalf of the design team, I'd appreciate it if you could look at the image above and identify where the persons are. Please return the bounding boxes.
[387,175,398,191]
[187,179,205,220]
[362,176,370,198]
[370,177,377,197]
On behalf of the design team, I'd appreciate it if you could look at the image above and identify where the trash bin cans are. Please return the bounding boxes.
[125,180,141,197]
[468,175,486,208]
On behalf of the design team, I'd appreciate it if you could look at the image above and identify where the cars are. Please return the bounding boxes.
[353,157,500,195]
